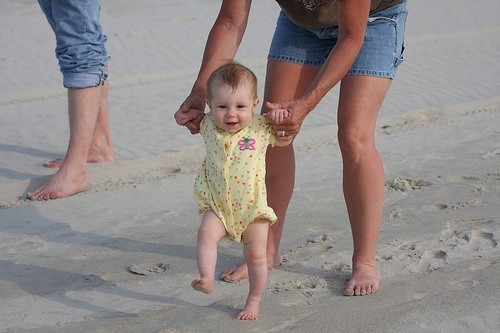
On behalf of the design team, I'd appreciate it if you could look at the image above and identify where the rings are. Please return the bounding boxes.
[281,130,285,137]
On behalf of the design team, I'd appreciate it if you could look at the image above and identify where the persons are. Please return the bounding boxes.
[176,62,293,321]
[175,0,409,298]
[25,0,114,200]
